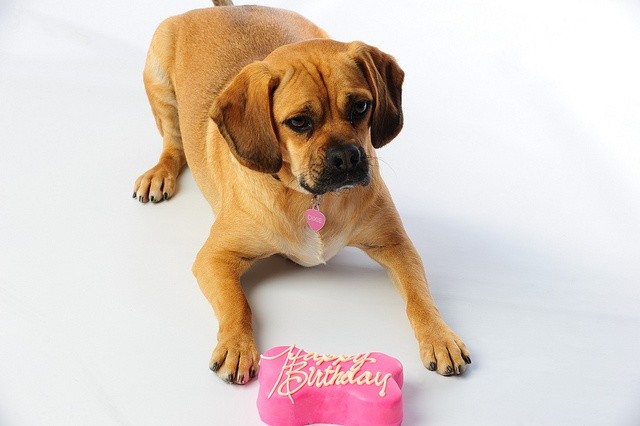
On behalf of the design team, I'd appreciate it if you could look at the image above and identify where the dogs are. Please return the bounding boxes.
[132,0,474,386]
